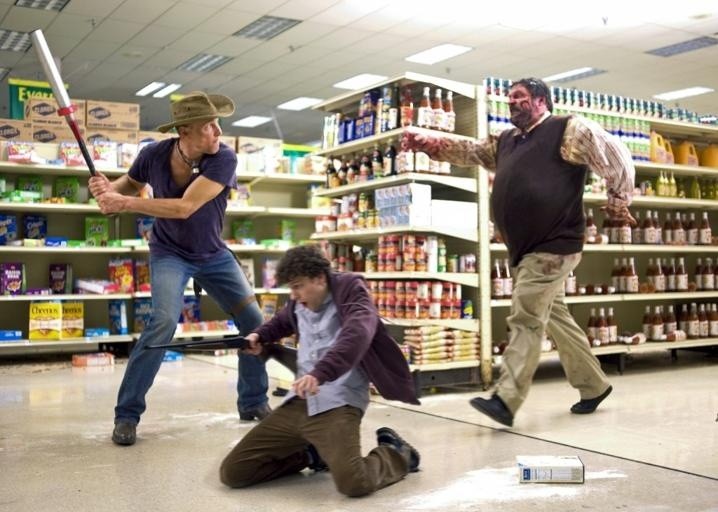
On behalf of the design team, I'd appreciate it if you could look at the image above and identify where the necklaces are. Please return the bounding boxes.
[177,140,202,176]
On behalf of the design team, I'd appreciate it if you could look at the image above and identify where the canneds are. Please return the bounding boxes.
[365,281,472,319]
[319,234,474,273]
[484,79,718,161]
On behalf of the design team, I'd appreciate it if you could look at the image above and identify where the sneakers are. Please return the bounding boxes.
[378,428,420,468]
[308,448,329,473]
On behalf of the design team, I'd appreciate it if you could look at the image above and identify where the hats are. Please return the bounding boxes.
[158,90,235,133]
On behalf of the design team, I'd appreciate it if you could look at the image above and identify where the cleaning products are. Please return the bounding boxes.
[650,130,666,163]
[660,138,674,164]
[701,141,718,168]
[677,140,700,166]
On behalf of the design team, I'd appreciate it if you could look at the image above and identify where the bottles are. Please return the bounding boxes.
[489,216,516,356]
[539,206,718,353]
[326,132,451,190]
[321,112,343,149]
[655,169,718,200]
[426,236,476,273]
[386,82,456,134]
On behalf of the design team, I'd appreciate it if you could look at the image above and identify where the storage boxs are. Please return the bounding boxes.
[0,97,171,145]
[238,136,283,158]
[219,137,236,152]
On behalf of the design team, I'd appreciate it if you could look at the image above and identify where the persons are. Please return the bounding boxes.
[221,245,421,496]
[87,94,271,446]
[400,79,639,427]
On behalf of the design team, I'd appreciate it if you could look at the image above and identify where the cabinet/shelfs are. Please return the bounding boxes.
[310,71,718,388]
[0,164,327,354]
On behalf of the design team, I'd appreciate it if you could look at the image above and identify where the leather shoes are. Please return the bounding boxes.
[114,423,137,446]
[570,385,612,414]
[471,395,513,427]
[239,405,272,420]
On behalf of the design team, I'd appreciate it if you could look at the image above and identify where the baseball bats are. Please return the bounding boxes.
[27,29,117,220]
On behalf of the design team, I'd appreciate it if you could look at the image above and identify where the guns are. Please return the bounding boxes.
[142,336,296,377]
